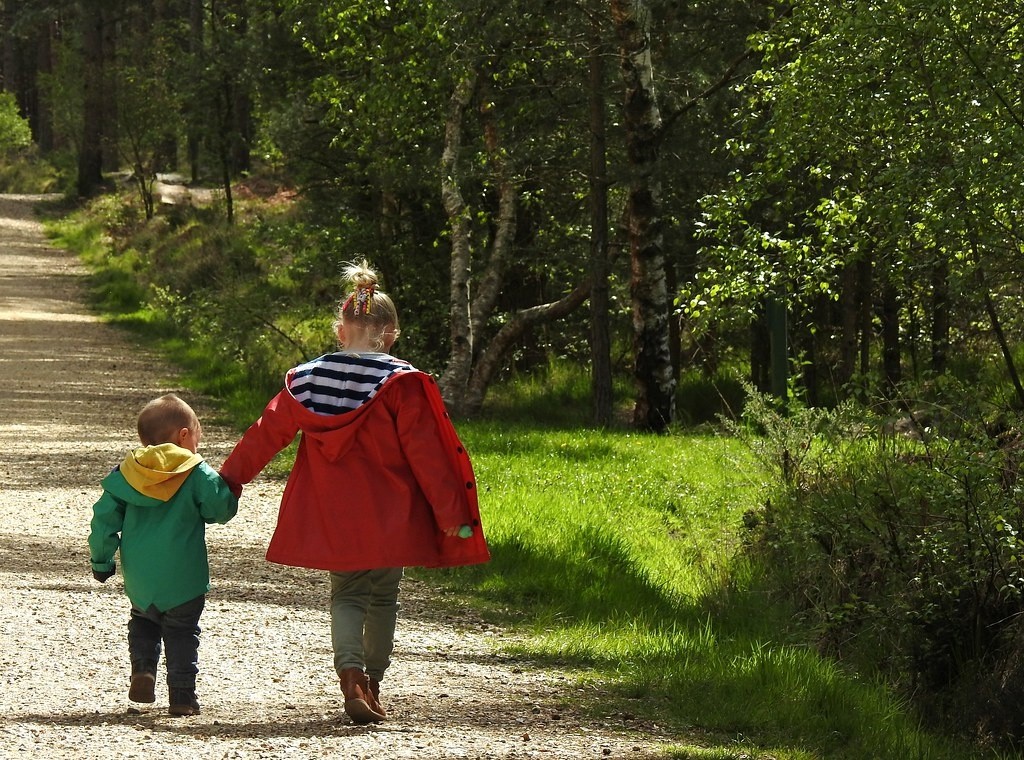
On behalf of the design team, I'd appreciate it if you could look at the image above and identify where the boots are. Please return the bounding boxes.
[339,667,388,721]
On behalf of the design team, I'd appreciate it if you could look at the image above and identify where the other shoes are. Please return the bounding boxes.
[168,687,200,714]
[129,660,156,703]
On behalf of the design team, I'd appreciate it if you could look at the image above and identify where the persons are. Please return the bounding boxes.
[85,391,237,716]
[217,254,491,722]
